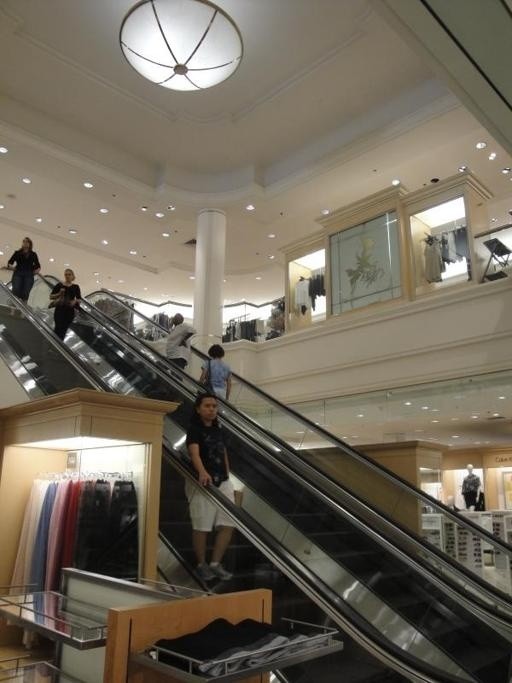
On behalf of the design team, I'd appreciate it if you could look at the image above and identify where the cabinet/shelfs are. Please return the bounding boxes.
[422,507,512,574]
[0,568,344,683]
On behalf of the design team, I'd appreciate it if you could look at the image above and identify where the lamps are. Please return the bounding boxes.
[118,0,244,91]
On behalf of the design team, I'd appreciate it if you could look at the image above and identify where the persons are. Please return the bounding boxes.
[165,312,197,370]
[458,462,481,506]
[180,392,239,581]
[49,267,83,340]
[8,236,42,304]
[194,343,232,400]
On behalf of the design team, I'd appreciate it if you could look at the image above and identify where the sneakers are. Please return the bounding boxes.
[210,562,234,581]
[197,562,215,579]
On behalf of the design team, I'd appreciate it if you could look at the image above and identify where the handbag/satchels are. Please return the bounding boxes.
[200,380,216,397]
[49,297,65,309]
